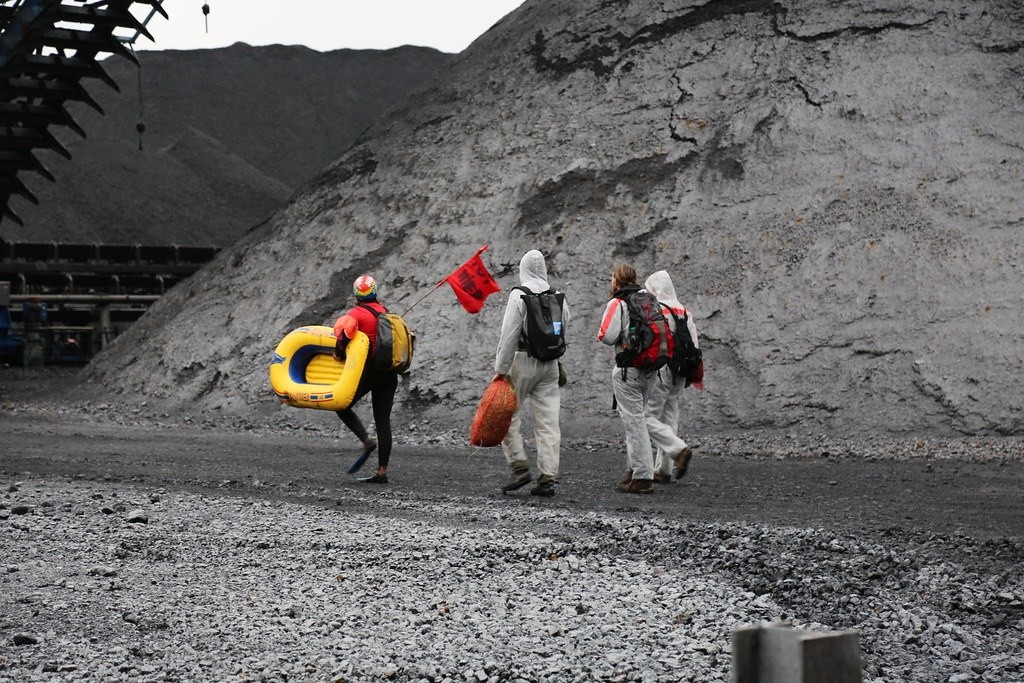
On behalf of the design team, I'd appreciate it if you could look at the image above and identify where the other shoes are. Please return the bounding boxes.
[675,448,692,479]
[654,473,671,483]
[615,473,653,494]
[531,486,554,498]
[502,470,531,490]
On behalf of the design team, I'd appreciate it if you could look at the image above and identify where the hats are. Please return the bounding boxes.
[353,275,377,297]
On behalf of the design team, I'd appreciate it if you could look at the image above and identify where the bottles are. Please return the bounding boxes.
[628,327,639,344]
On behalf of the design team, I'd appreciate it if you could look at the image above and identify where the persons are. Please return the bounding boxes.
[335,276,398,487]
[490,249,571,498]
[643,270,698,486]
[596,263,691,495]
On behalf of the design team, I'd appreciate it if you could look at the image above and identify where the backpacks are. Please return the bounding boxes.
[612,290,673,380]
[512,284,566,361]
[659,301,702,388]
[360,305,413,374]
